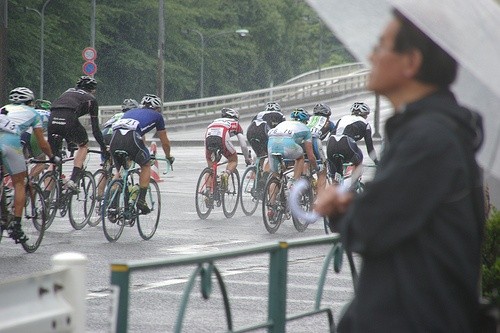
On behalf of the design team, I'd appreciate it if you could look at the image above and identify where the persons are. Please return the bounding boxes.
[205,107,251,209]
[0,87,61,243]
[247,102,286,180]
[28,98,52,177]
[300,103,335,198]
[108,93,172,214]
[267,108,318,218]
[317,7,485,333]
[327,102,377,191]
[47,76,111,200]
[97,98,139,202]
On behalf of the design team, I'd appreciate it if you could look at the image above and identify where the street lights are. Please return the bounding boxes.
[15,0,52,100]
[179,28,251,99]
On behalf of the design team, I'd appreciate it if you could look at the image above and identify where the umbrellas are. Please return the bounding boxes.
[287,0,500,223]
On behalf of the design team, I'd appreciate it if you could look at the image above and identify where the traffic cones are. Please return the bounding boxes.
[147,142,164,182]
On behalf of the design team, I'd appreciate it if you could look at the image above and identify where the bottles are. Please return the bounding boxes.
[283,183,289,198]
[62,173,68,189]
[3,186,12,210]
[217,175,221,187]
[127,182,132,200]
[131,183,139,201]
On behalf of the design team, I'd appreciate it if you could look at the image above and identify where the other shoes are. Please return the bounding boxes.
[67,180,80,192]
[7,220,26,240]
[107,204,116,223]
[96,202,103,215]
[220,170,229,191]
[204,194,214,209]
[136,198,150,214]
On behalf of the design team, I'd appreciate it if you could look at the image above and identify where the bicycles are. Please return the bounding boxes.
[0,139,65,254]
[100,150,175,242]
[195,148,252,220]
[30,134,111,232]
[239,154,377,234]
[83,151,116,227]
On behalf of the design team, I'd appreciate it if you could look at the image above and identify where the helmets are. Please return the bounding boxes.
[140,94,163,109]
[33,98,52,109]
[350,102,370,117]
[8,87,35,103]
[290,108,309,121]
[312,103,332,116]
[265,101,282,112]
[76,76,97,90]
[122,98,139,110]
[221,106,239,119]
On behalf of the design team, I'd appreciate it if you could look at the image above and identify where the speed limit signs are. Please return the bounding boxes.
[82,47,98,62]
